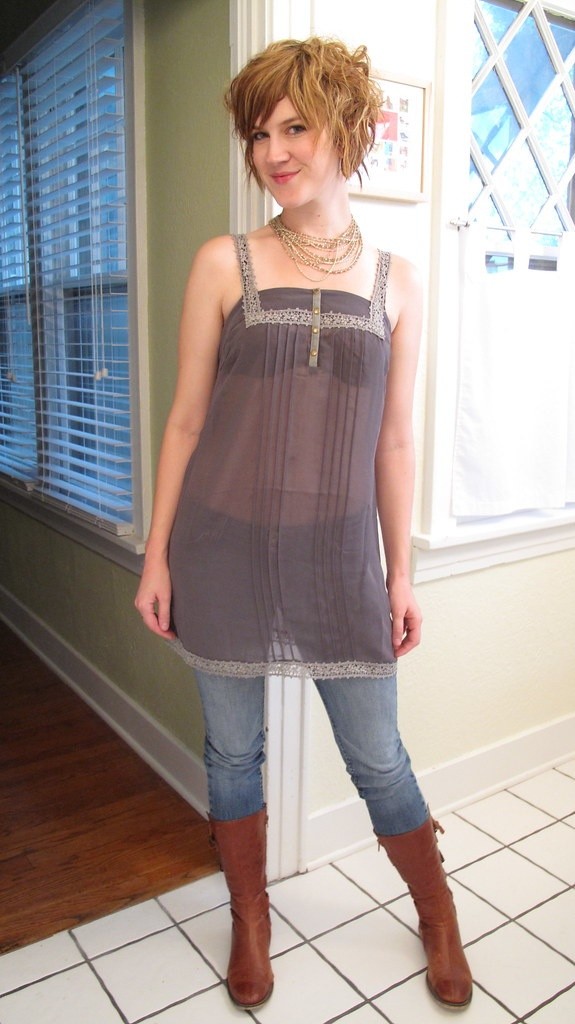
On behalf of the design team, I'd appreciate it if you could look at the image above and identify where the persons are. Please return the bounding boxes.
[136,37,474,1010]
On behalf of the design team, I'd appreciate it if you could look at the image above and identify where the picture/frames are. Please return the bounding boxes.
[347,69,432,204]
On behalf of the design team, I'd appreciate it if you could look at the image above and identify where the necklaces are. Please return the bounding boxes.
[270,215,362,282]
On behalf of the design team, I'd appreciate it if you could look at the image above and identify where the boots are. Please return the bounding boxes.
[206,803,274,1009]
[373,807,473,1011]
